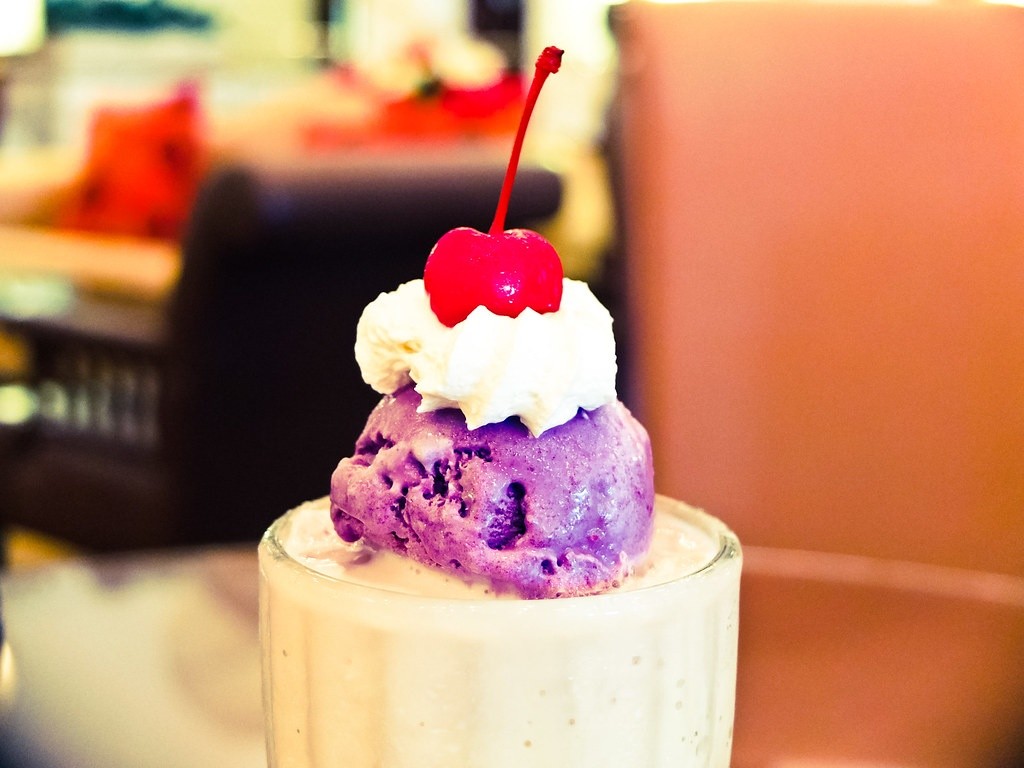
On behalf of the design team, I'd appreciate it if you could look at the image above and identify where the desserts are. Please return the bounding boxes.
[260,44,743,767]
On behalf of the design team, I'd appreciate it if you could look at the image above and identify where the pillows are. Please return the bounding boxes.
[370,76,522,150]
[53,78,207,245]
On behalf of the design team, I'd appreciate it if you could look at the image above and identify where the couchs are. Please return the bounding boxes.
[0,52,615,350]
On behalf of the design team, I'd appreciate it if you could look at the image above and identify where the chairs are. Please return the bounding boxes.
[613,0,1024,768]
[0,149,566,588]
